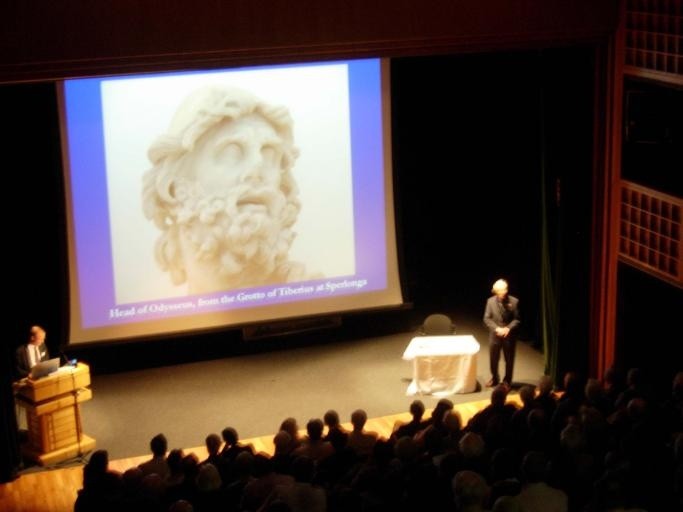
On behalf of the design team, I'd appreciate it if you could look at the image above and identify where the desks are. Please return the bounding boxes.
[402,335,480,398]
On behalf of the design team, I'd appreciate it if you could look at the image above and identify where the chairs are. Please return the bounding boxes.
[416,314,455,334]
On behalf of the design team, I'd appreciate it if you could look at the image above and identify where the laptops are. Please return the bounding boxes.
[31,357,60,380]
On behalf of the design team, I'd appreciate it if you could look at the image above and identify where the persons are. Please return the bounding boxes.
[139,82,300,290]
[11,325,50,377]
[483,279,521,389]
[69,360,683,511]
